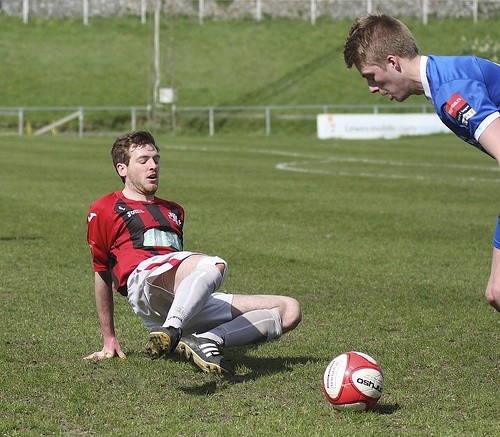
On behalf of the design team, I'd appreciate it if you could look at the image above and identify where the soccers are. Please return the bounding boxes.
[322,351,384,415]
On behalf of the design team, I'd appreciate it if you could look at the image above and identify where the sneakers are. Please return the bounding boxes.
[178,331,235,376]
[145,325,183,359]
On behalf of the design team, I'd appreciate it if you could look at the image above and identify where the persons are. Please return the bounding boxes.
[86,129,301,376]
[343,15,500,311]
